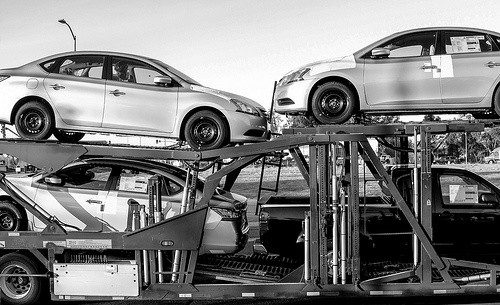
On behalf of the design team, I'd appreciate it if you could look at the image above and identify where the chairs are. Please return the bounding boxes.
[112,61,134,84]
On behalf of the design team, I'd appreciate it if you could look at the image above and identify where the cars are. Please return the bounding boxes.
[0,50,271,150]
[273,25,500,126]
[0,158,248,264]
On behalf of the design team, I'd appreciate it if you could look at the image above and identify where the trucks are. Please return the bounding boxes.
[483,147,500,164]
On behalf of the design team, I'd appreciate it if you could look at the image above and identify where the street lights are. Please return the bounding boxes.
[58,19,76,53]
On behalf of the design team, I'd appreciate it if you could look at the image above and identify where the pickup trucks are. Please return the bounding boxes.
[258,162,500,284]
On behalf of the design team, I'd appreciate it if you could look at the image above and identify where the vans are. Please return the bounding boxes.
[445,156,460,164]
